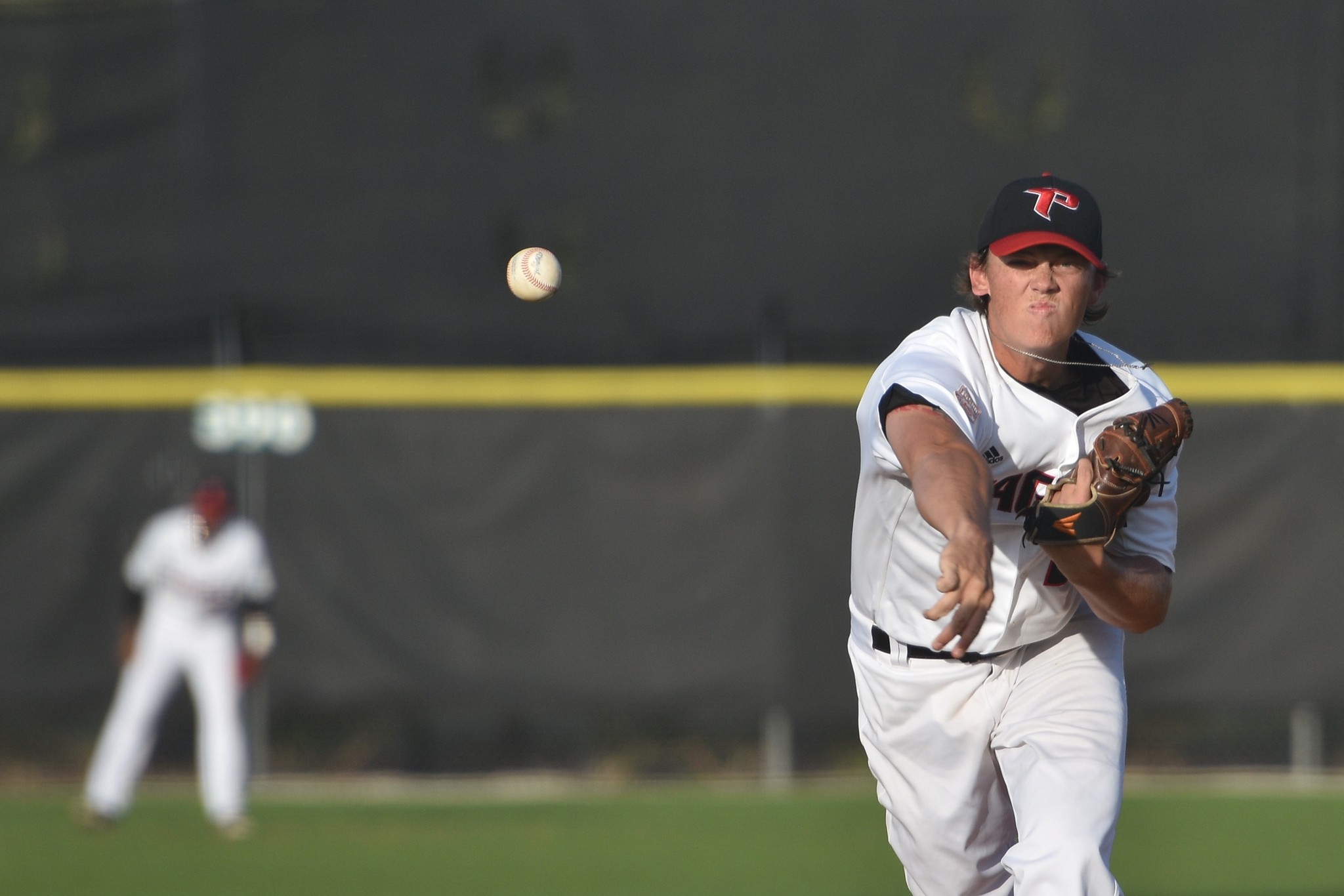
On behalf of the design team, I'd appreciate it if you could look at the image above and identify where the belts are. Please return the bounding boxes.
[871,626,1005,663]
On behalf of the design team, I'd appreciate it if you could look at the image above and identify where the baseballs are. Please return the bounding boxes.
[505,247,563,304]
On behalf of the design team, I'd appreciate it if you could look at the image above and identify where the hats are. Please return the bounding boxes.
[976,172,1106,271]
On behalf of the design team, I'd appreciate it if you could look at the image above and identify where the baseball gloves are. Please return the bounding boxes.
[1014,395,1195,552]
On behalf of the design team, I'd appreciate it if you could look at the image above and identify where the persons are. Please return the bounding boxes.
[849,170,1185,896]
[78,475,280,841]
